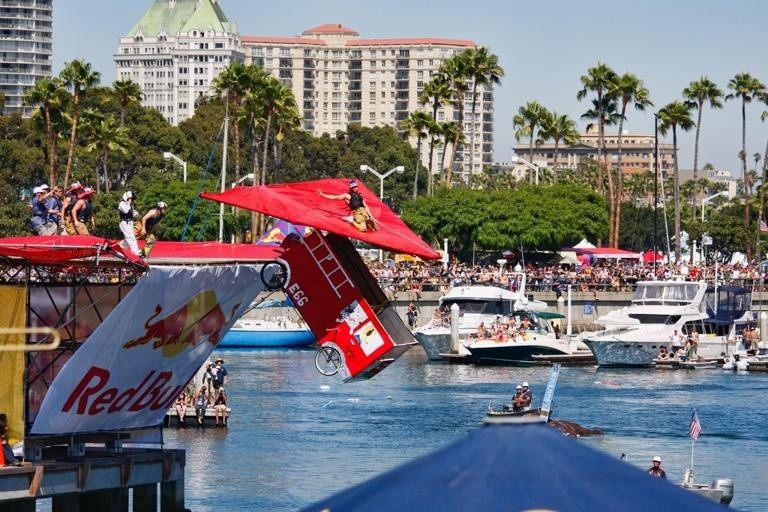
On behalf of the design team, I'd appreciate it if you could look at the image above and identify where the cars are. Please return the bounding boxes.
[453,250,566,271]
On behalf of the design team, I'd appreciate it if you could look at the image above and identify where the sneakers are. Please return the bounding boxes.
[342,215,353,220]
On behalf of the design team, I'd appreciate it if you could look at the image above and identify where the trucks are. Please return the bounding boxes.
[394,248,448,267]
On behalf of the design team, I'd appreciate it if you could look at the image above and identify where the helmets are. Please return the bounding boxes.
[651,454,662,464]
[522,381,530,387]
[515,384,522,389]
[346,179,359,189]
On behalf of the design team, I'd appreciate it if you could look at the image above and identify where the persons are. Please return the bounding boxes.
[139,202,168,246]
[318,180,378,232]
[27,182,97,236]
[510,385,530,412]
[522,382,532,411]
[646,456,667,480]
[0,414,18,466]
[118,191,143,257]
[175,357,228,426]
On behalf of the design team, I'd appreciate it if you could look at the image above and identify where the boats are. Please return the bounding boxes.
[480,403,555,425]
[700,190,731,271]
[213,313,316,348]
[617,455,734,506]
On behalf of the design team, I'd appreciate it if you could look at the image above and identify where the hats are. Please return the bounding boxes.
[121,191,137,201]
[218,387,224,392]
[63,182,83,196]
[75,187,96,200]
[33,187,46,194]
[215,357,225,364]
[40,184,51,189]
[157,201,168,208]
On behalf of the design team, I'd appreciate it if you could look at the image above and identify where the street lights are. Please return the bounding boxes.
[230,173,256,245]
[359,162,408,266]
[163,152,187,186]
[511,155,539,187]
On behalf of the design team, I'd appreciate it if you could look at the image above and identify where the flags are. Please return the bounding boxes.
[689,410,702,443]
[759,220,768,232]
